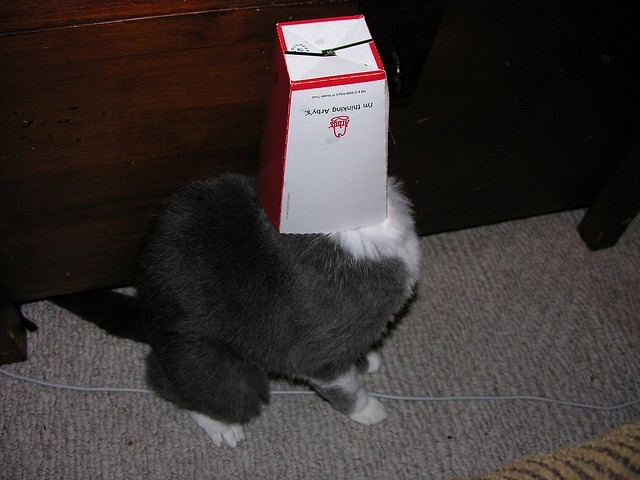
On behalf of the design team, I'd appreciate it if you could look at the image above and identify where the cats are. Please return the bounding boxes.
[47,164,424,451]
